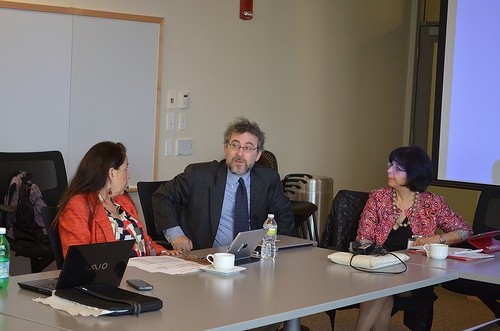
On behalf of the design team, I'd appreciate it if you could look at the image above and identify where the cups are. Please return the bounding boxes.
[423,243,449,259]
[207,252,235,271]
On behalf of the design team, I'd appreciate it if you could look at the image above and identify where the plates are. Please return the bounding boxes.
[199,265,247,275]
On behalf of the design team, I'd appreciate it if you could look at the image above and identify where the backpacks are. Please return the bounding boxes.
[0,170,52,260]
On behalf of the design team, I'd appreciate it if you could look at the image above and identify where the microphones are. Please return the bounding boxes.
[349,241,388,255]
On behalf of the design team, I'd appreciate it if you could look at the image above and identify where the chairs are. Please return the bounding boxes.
[319,189,438,331]
[41,206,64,271]
[137,181,188,251]
[441,190,500,319]
[0,151,68,273]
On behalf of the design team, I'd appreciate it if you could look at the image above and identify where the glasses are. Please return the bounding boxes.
[227,140,258,153]
[387,161,407,173]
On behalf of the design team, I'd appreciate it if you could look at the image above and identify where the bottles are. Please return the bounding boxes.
[261,213,278,258]
[0,227,11,288]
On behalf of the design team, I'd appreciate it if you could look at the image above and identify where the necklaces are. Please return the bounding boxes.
[392,188,419,227]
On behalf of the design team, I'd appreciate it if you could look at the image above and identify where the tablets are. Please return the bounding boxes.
[467,229,500,240]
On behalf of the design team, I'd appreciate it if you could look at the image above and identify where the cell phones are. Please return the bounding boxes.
[126,277,153,290]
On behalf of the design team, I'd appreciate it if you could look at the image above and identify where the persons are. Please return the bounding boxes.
[51,141,183,257]
[152,118,295,331]
[352,146,473,331]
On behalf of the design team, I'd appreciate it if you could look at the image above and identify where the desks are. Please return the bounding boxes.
[0,229,500,331]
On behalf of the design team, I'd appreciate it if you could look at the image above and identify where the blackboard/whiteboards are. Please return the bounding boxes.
[0,0,165,193]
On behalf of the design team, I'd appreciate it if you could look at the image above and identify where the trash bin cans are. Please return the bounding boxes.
[285,175,334,244]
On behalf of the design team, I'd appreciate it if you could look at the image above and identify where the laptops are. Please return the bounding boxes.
[16,239,136,297]
[193,227,271,261]
[256,234,318,249]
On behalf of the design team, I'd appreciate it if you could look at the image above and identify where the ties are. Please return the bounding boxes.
[233,177,250,241]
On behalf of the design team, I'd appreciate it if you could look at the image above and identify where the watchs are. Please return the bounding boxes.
[439,234,446,244]
